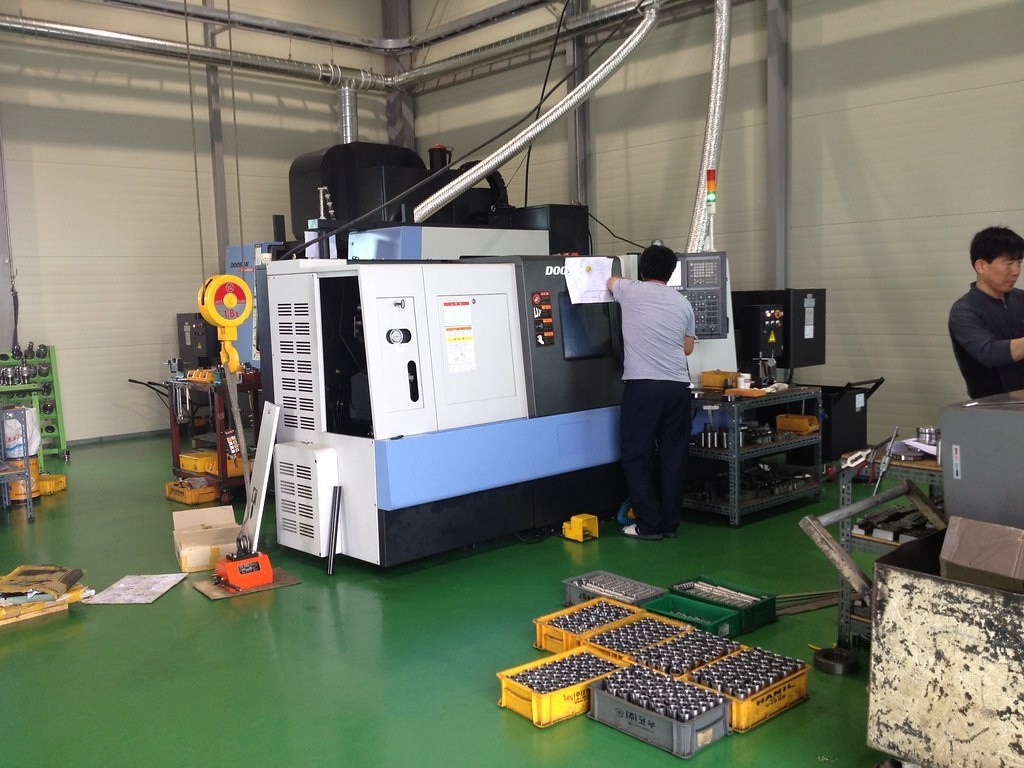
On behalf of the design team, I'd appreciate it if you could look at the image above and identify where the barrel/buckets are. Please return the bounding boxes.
[3,408,39,458]
[5,455,41,504]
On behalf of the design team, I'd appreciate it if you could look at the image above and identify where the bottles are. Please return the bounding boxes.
[36,344,47,358]
[43,404,52,414]
[25,341,34,359]
[42,383,50,395]
[12,344,22,360]
[39,363,49,376]
[28,364,37,378]
[21,358,29,384]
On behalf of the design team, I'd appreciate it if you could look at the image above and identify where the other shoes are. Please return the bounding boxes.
[663,531,677,538]
[618,523,663,540]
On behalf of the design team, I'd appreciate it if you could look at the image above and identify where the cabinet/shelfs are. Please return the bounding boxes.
[837,438,944,648]
[679,383,822,529]
[0,407,36,522]
[167,369,262,506]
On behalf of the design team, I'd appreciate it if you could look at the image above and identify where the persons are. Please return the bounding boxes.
[948,225,1024,401]
[606,244,698,540]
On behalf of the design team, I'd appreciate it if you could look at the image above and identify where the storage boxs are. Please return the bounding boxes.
[493,644,625,729]
[171,506,242,572]
[164,481,214,504]
[666,574,777,633]
[940,517,1024,595]
[702,370,742,390]
[582,612,694,659]
[531,596,647,654]
[177,450,251,478]
[621,628,748,680]
[560,569,669,609]
[776,415,818,431]
[638,592,742,639]
[677,645,811,734]
[585,663,733,760]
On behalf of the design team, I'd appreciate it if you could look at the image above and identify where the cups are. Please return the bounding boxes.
[5,367,15,385]
[698,431,745,449]
[741,374,751,388]
[428,148,452,170]
